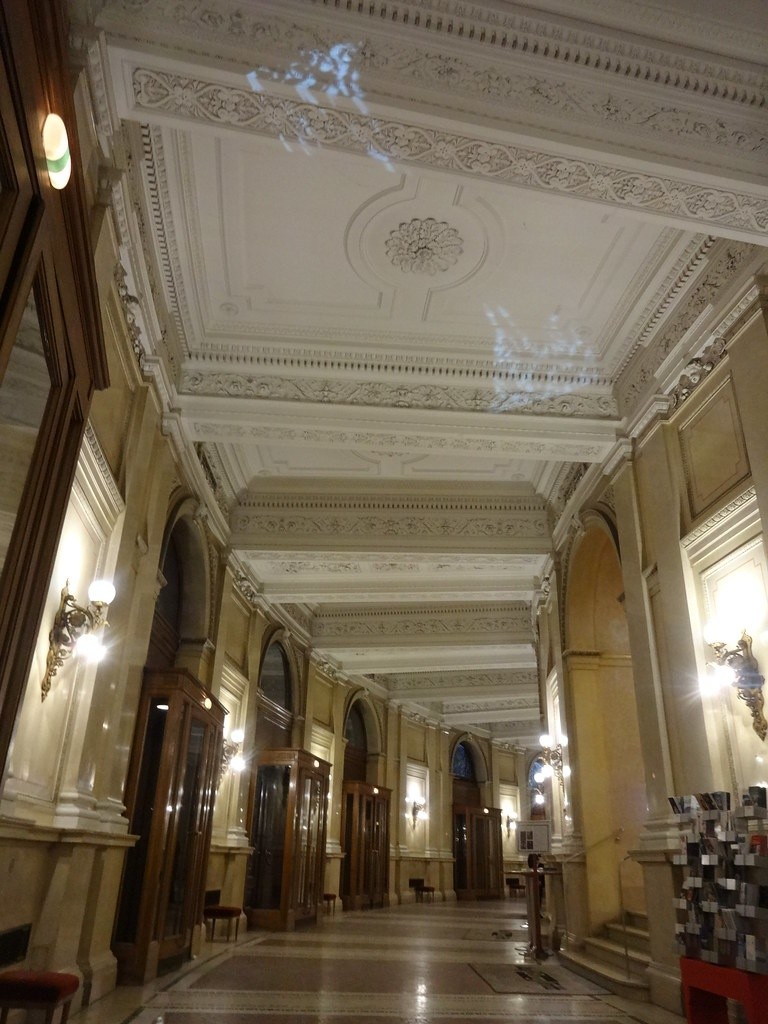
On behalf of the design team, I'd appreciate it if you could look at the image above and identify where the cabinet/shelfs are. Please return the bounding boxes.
[667,786,768,1024]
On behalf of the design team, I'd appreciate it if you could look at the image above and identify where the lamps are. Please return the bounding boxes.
[41,576,117,704]
[702,616,768,741]
[216,729,245,790]
[539,733,565,794]
[42,111,73,190]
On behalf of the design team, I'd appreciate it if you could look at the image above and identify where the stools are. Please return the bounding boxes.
[204,904,241,943]
[322,893,337,915]
[0,969,80,1024]
[415,887,435,903]
